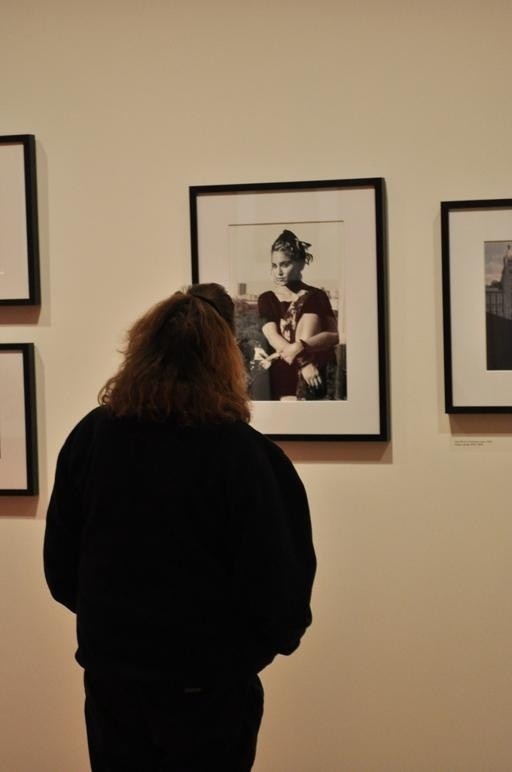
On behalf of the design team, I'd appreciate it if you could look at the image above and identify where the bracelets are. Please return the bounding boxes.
[295,352,314,368]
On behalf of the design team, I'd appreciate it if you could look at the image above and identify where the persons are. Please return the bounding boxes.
[253,227,341,400]
[40,284,318,771]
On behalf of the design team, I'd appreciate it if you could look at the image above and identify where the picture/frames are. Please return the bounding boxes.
[0,343,39,496]
[188,177,388,443]
[0,134,41,306]
[441,198,512,414]
